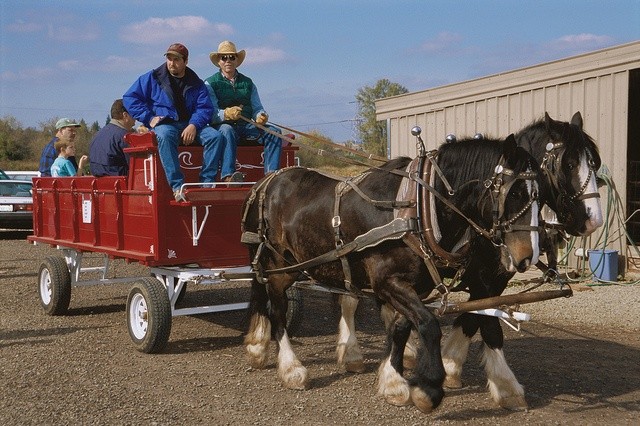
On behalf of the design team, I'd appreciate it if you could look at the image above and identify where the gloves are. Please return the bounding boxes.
[224,106,242,121]
[256,112,268,125]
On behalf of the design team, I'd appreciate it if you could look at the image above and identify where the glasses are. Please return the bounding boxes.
[221,55,235,61]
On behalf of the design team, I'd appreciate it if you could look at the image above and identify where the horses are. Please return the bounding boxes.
[239,133,541,415]
[334,110,604,412]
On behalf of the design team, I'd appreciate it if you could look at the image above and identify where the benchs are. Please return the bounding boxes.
[124,133,299,189]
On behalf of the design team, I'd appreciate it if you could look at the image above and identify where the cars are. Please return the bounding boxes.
[2,171,41,179]
[0,179,32,238]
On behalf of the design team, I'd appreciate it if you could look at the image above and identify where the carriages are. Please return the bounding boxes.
[26,102,603,415]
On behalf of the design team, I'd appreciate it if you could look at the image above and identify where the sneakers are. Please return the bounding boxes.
[224,172,243,188]
[173,188,187,202]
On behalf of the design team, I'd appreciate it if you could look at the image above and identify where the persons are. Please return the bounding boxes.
[49,137,88,177]
[122,42,224,201]
[40,117,85,176]
[88,99,136,177]
[204,41,283,187]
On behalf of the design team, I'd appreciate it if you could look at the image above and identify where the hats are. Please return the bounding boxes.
[55,118,81,130]
[164,43,188,58]
[209,40,245,67]
